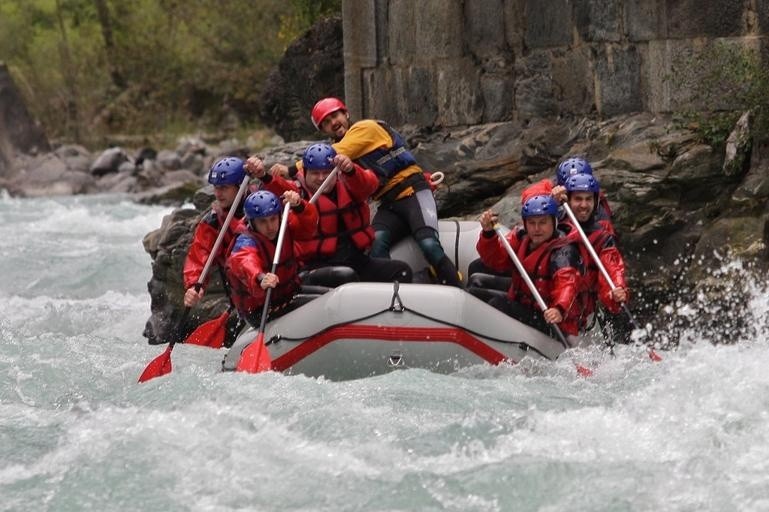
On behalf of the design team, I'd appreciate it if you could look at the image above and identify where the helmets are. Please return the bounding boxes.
[522,195,558,218]
[243,190,282,218]
[556,158,599,194]
[302,145,336,169]
[311,98,347,130]
[207,156,246,184]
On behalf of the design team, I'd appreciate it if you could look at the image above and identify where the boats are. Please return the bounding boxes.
[217,221,579,382]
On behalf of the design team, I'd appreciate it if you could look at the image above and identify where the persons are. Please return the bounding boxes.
[469,196,584,345]
[183,155,301,306]
[298,143,412,283]
[535,158,610,232]
[551,174,627,323]
[304,97,465,289]
[224,190,356,328]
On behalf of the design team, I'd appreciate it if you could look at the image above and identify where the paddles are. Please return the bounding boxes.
[137,166,251,382]
[187,164,343,348]
[237,193,291,372]
[490,218,592,378]
[563,196,663,361]
